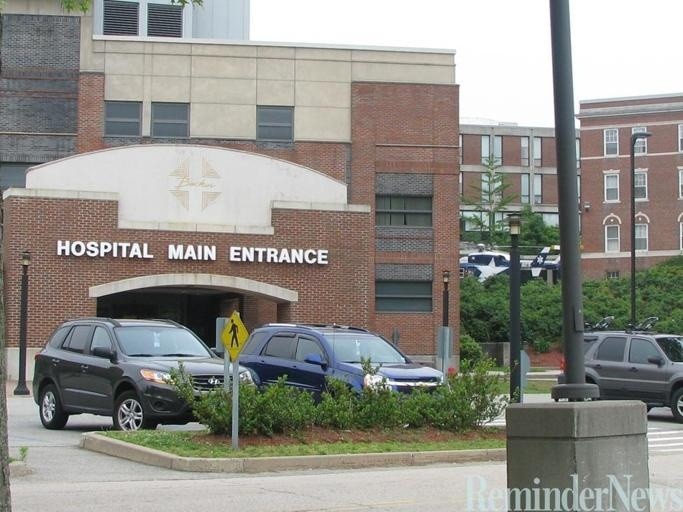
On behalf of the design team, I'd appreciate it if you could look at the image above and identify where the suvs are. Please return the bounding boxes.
[32,317,260,433]
[561,329,683,423]
[232,321,450,411]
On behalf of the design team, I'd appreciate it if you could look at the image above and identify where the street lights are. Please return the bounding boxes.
[629,131,652,331]
[440,268,450,380]
[505,210,524,403]
[13,250,31,395]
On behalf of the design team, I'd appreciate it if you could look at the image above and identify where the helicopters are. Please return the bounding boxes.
[459,244,560,286]
